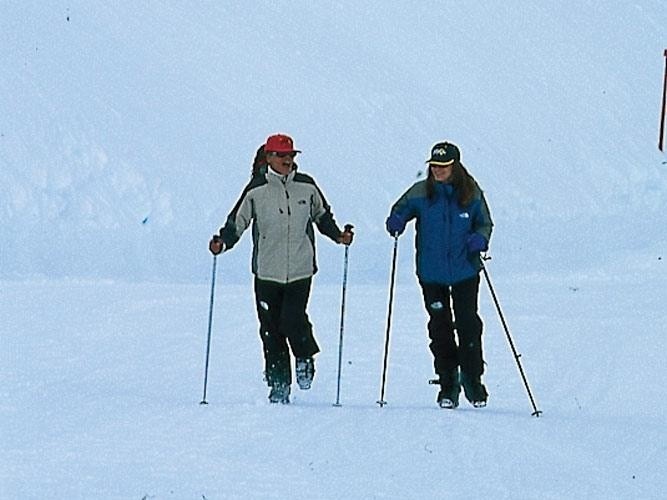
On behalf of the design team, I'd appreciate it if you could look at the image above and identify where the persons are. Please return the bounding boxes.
[208,131,353,405]
[382,141,494,411]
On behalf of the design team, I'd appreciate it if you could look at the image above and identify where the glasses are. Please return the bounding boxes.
[269,151,297,158]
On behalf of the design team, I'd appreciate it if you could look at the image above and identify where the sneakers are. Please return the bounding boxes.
[267,382,292,402]
[437,383,488,408]
[294,355,315,387]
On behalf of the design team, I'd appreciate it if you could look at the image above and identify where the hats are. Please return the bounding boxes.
[262,133,302,153]
[424,141,461,167]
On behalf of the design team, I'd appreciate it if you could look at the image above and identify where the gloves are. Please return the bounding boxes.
[385,214,406,238]
[465,230,489,254]
